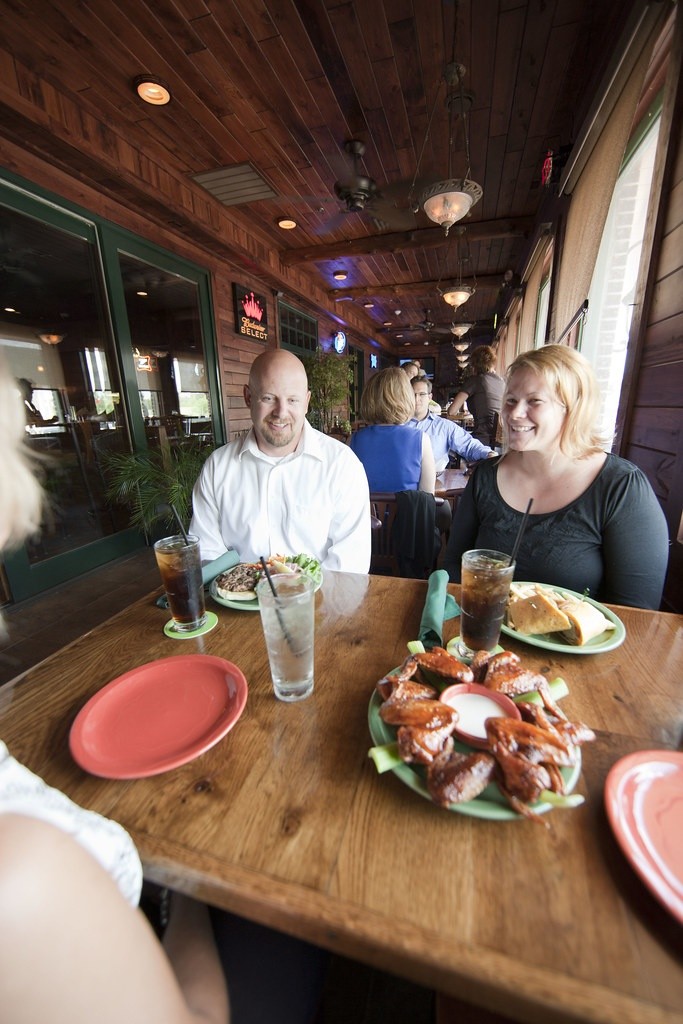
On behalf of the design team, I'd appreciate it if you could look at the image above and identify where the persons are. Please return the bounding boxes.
[187,348,371,575]
[0,367,367,1024]
[17,378,149,428]
[346,344,505,557]
[439,344,670,612]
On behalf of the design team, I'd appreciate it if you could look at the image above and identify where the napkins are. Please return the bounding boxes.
[418,569,461,649]
[155,549,240,609]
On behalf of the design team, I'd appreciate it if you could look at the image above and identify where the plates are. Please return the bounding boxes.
[604,750,683,923]
[368,659,581,821]
[501,581,626,654]
[69,655,248,779]
[210,564,324,610]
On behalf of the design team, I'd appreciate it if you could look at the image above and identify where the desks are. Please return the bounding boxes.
[28,420,118,539]
[1,558,683,1024]
[442,412,473,430]
[435,468,470,517]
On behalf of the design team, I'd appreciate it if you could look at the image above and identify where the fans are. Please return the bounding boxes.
[276,140,415,235]
[410,309,450,334]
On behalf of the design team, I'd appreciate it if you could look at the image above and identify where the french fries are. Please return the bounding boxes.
[505,583,580,638]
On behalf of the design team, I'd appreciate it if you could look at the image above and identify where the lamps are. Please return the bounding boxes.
[31,328,67,348]
[277,216,298,230]
[333,271,347,280]
[406,63,484,238]
[437,216,479,312]
[451,323,472,371]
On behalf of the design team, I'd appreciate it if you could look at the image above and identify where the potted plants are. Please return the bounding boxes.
[95,424,215,534]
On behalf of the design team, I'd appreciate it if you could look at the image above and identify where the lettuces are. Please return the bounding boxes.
[283,553,321,593]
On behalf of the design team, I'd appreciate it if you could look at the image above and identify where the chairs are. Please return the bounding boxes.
[369,493,443,580]
[325,432,350,443]
[489,412,502,455]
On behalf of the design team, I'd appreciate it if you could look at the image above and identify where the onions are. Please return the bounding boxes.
[260,562,304,577]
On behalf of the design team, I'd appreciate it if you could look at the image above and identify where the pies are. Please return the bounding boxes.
[506,594,610,646]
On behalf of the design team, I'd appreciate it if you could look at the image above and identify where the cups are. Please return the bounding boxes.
[67,405,77,422]
[459,549,516,660]
[257,573,316,702]
[153,535,207,634]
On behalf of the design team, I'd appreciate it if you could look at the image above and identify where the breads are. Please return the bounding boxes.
[214,564,259,601]
[272,560,292,573]
